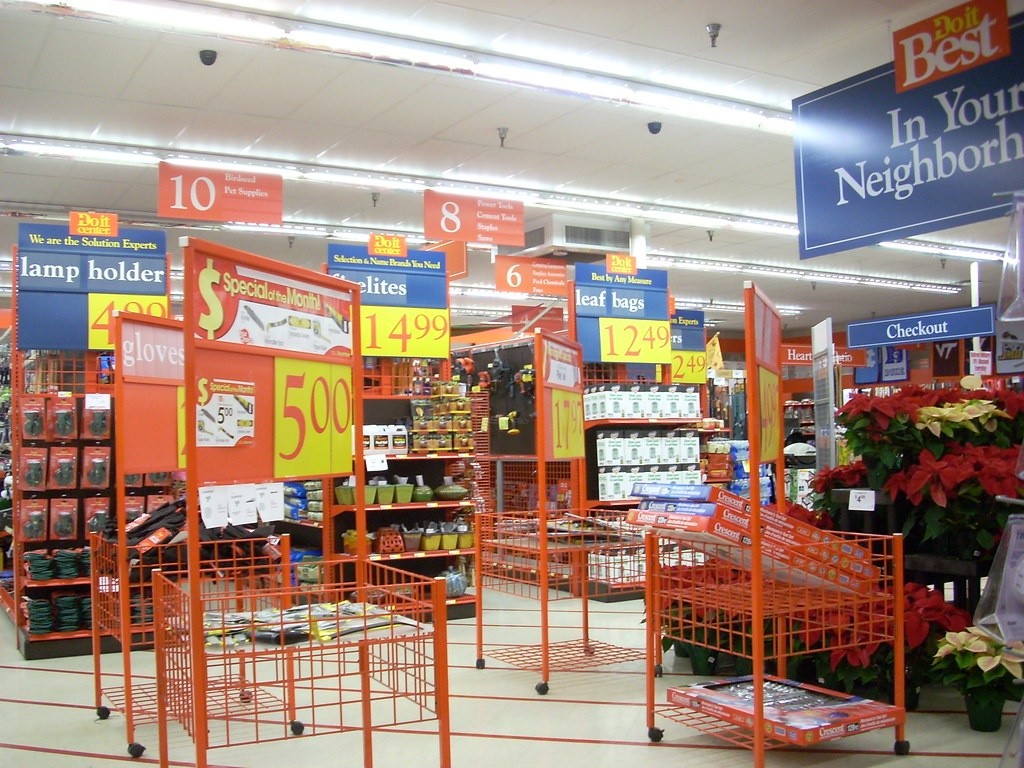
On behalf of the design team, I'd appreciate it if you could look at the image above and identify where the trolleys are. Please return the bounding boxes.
[645,279,911,767]
[474,326,664,695]
[89,311,304,736]
[151,236,451,768]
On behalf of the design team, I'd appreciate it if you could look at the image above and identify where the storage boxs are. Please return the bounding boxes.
[582,384,705,584]
[666,673,905,748]
[393,381,474,455]
[625,483,880,594]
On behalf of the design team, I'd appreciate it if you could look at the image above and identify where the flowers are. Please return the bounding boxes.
[639,556,972,692]
[766,385,1024,560]
[929,626,1024,703]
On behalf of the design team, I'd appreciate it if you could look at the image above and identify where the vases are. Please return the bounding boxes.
[964,691,1006,732]
[862,454,897,491]
[888,673,921,711]
[674,639,817,682]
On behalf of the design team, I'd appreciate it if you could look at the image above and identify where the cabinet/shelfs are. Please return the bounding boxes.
[0,315,732,661]
